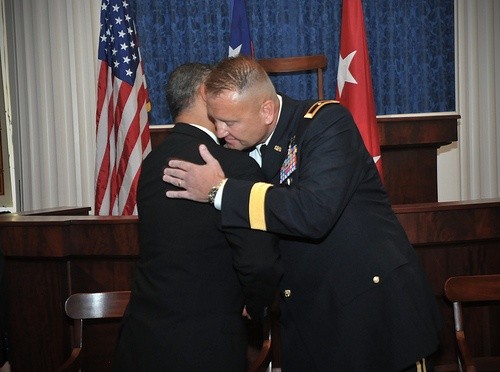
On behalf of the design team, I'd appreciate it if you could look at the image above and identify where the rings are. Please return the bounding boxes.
[178,179,181,188]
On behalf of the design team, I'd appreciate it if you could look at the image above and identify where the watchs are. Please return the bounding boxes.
[208,178,225,206]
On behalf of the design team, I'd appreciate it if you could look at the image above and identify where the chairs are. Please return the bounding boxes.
[245,319,272,372]
[258,55,328,101]
[445,275,500,372]
[57,290,131,372]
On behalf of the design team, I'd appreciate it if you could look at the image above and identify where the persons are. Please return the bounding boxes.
[111,62,285,372]
[162,56,446,372]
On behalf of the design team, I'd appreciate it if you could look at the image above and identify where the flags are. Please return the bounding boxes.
[336,1,384,186]
[227,0,255,59]
[94,0,153,217]
